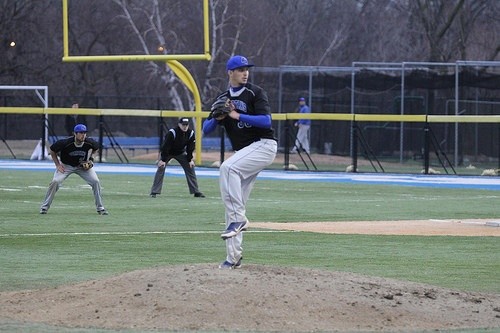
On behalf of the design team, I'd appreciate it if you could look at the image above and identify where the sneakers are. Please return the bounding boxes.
[220,219,250,240]
[219,257,242,269]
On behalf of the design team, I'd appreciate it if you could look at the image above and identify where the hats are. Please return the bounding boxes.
[226,55,255,70]
[299,97,305,101]
[179,117,190,125]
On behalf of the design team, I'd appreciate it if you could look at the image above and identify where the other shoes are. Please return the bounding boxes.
[290,150,299,154]
[194,193,205,197]
[150,193,157,198]
[40,208,47,214]
[98,210,108,215]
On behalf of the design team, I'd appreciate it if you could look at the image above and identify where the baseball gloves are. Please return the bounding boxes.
[80,161,94,171]
[208,95,236,119]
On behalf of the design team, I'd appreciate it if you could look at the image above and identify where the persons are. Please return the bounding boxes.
[39,124,109,215]
[203,55,277,269]
[65,102,88,136]
[150,117,206,198]
[290,98,311,154]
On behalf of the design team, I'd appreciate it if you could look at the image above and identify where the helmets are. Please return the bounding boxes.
[74,124,88,133]
